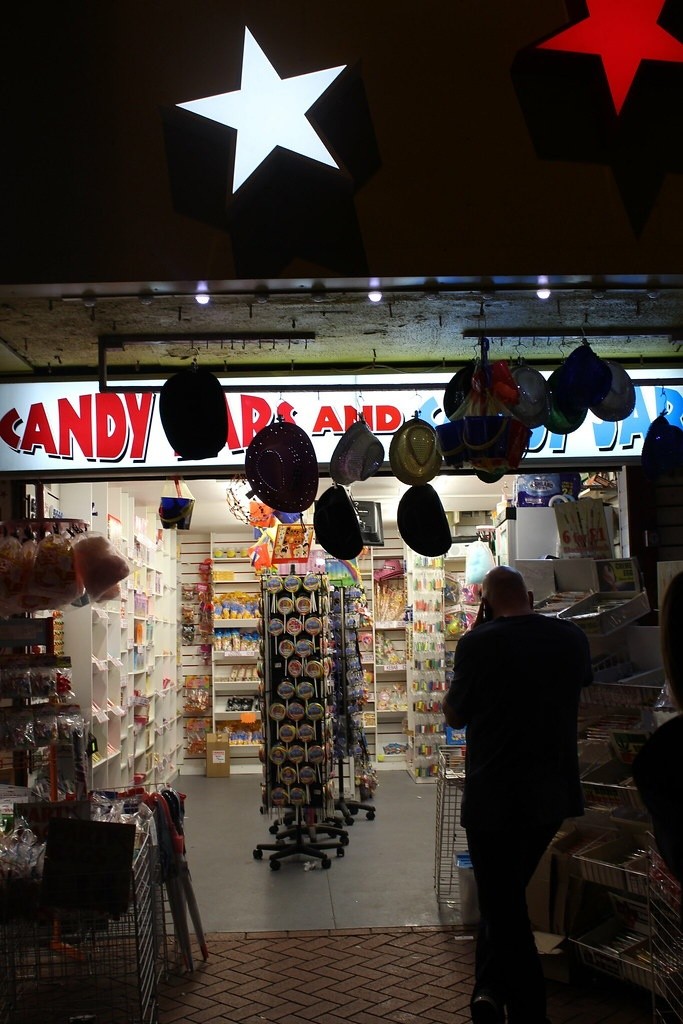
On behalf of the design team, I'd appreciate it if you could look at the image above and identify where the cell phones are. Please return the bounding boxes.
[473,601,484,628]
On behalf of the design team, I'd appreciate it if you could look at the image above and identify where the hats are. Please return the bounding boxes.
[313,485,363,559]
[331,420,385,486]
[397,484,453,557]
[158,366,228,458]
[443,345,636,435]
[390,419,443,486]
[245,422,321,513]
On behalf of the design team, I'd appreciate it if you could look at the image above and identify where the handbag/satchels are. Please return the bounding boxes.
[436,391,534,483]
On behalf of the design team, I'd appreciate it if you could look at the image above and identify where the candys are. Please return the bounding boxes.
[266,572,324,825]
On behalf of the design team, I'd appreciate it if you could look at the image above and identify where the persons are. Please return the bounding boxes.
[445,566,595,1024]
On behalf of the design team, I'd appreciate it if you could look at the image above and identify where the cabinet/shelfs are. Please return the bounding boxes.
[212,556,263,748]
[552,660,683,1000]
[358,543,412,766]
[58,481,182,799]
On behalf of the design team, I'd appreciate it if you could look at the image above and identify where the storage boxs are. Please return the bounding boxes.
[455,848,481,924]
[205,732,232,776]
[535,589,679,999]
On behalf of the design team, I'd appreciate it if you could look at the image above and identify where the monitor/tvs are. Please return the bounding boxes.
[315,500,385,547]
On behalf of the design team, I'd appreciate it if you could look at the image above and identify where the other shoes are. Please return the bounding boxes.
[470,995,504,1024]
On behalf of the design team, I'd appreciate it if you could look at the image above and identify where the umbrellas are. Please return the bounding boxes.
[148,787,209,973]
